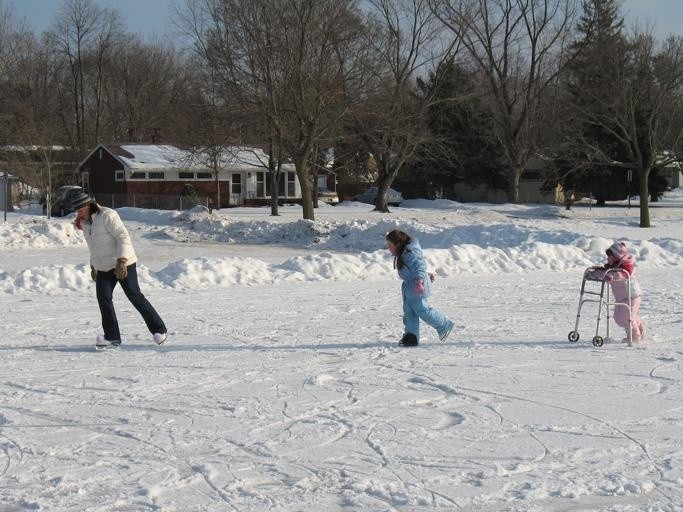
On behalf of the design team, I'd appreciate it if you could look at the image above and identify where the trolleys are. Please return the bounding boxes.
[567,266,633,349]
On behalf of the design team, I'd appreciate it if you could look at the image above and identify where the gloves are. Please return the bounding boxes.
[91,266,97,281]
[415,280,424,293]
[113,258,128,280]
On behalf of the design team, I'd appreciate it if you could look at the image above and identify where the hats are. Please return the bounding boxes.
[71,190,93,210]
[610,242,627,260]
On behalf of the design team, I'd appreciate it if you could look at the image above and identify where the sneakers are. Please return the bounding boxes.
[399,333,420,348]
[96,338,121,346]
[153,332,167,344]
[622,336,645,342]
[438,319,454,341]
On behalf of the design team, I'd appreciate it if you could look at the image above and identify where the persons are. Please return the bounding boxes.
[69,190,167,350]
[588,241,645,341]
[386,230,454,346]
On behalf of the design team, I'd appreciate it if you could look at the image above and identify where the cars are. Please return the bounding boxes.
[42,185,93,217]
[350,185,404,207]
[313,186,339,206]
